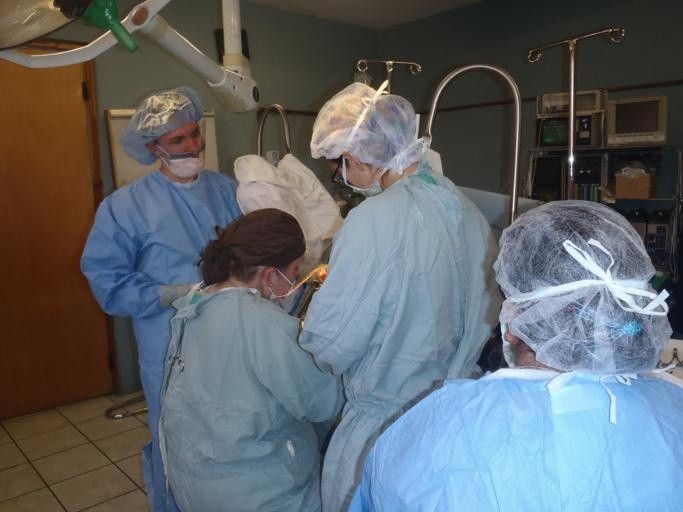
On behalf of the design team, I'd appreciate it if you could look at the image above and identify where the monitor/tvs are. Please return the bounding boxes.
[607,95,668,146]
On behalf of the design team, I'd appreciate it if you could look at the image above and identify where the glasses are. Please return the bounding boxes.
[329,160,344,185]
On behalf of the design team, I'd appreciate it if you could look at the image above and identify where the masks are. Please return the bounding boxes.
[264,267,295,305]
[154,140,206,178]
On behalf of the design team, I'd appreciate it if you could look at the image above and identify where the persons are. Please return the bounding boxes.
[79,87,242,512]
[347,198,683,512]
[297,81,503,511]
[151,207,345,512]
